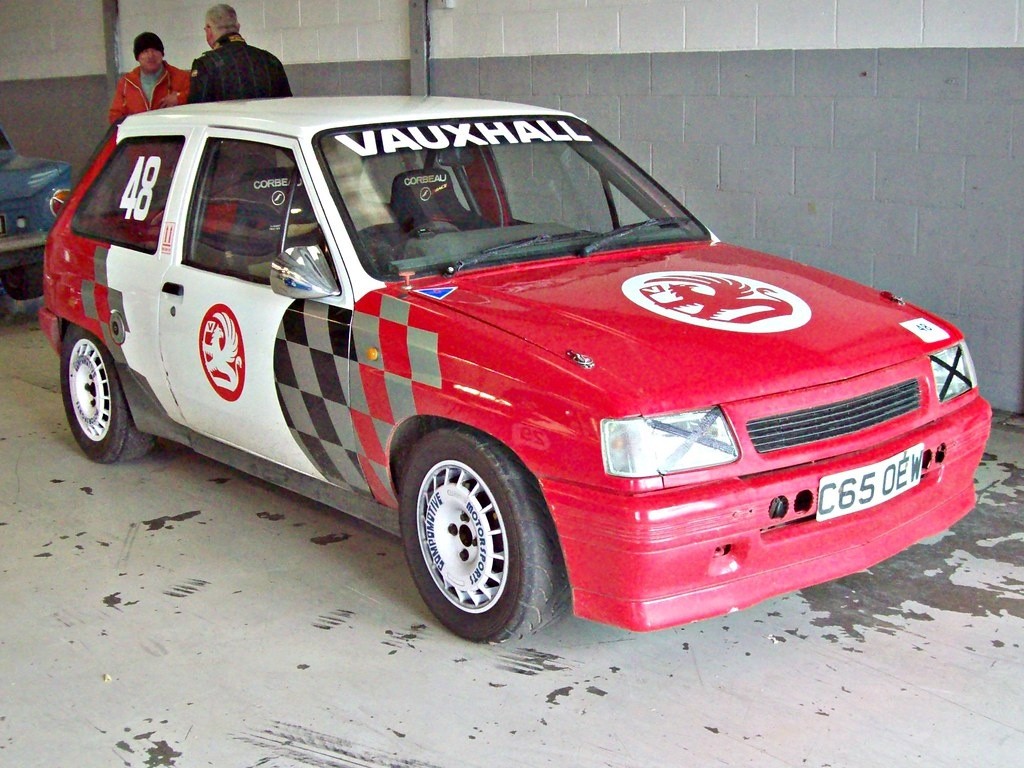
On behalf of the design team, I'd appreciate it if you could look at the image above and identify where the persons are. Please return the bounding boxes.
[107,31,191,126]
[189,4,293,104]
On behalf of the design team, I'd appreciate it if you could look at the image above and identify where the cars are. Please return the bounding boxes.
[0,134,77,299]
[40,92,996,648]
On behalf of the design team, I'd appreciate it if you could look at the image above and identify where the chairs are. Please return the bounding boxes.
[238,167,314,273]
[390,169,496,237]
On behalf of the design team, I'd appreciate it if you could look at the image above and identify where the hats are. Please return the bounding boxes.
[134,32,164,61]
